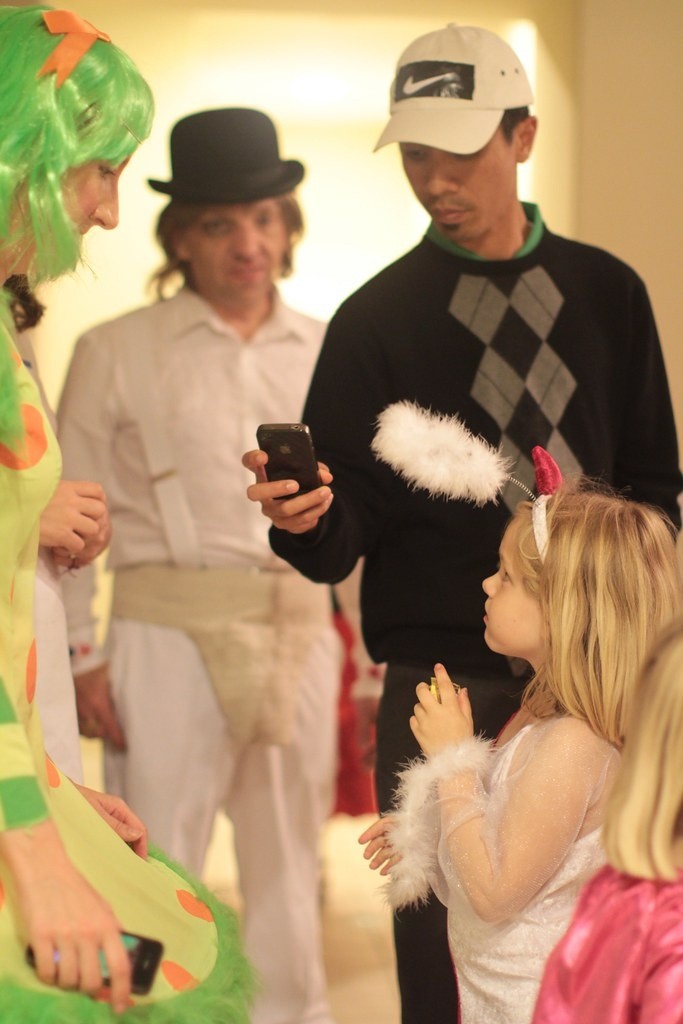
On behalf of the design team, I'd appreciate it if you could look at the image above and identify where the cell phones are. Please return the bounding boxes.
[258,422,323,500]
[26,930,163,997]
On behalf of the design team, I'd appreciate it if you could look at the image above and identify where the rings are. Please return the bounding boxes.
[84,720,98,732]
[70,554,76,559]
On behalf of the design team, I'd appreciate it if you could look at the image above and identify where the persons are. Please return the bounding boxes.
[1,6,258,1024]
[2,274,114,787]
[359,487,683,1024]
[56,108,344,1023]
[242,24,683,1024]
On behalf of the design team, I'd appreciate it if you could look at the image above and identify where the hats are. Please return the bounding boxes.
[147,108,305,202]
[373,23,534,155]
